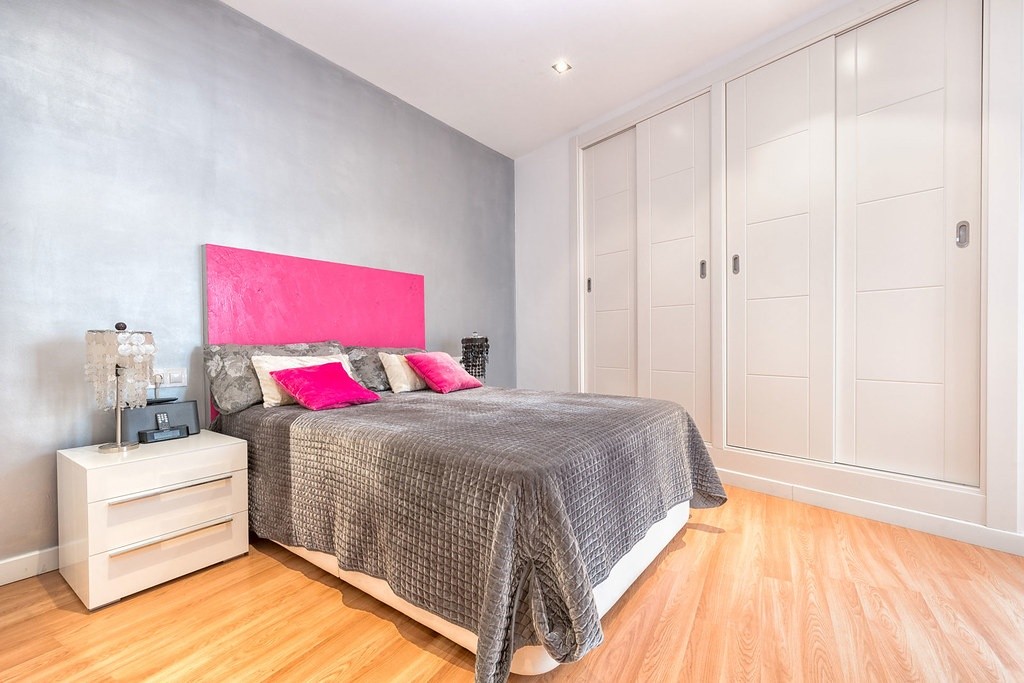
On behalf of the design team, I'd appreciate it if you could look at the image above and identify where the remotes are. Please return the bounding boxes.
[155,412,171,430]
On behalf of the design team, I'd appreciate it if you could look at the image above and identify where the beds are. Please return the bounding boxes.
[203,244,690,683]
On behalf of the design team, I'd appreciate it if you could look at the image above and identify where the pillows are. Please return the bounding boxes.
[404,351,484,394]
[378,352,430,393]
[269,362,381,411]
[344,346,428,392]
[251,354,355,409]
[203,340,366,416]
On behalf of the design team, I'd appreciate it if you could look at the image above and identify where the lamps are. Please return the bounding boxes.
[85,322,155,454]
[460,331,490,385]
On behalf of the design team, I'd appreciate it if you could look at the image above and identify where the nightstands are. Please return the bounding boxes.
[57,428,248,612]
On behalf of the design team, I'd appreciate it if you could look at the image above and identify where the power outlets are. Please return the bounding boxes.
[144,369,187,389]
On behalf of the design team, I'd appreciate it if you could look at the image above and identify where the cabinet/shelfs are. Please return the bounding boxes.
[566,0,988,527]
